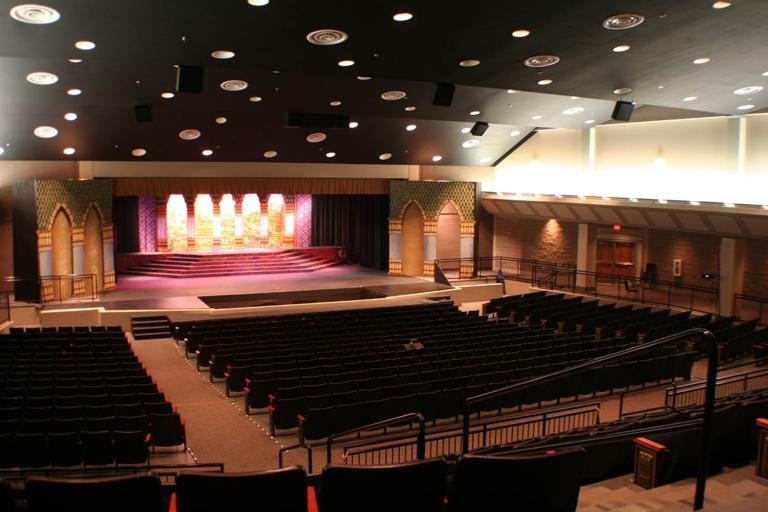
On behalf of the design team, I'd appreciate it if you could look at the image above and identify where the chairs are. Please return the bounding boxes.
[622,280,641,298]
[4,326,187,467]
[480,292,768,380]
[170,300,698,442]
[640,262,658,284]
[1,384,766,512]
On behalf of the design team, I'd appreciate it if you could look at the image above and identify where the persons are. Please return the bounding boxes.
[496,269,504,283]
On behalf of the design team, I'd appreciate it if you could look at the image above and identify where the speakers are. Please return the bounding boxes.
[610,99,634,122]
[432,82,456,107]
[175,63,205,95]
[470,121,489,138]
[133,103,152,124]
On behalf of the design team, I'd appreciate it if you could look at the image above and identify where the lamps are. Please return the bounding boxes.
[131,103,155,124]
[432,80,457,109]
[173,62,207,96]
[468,119,490,138]
[610,100,635,125]
